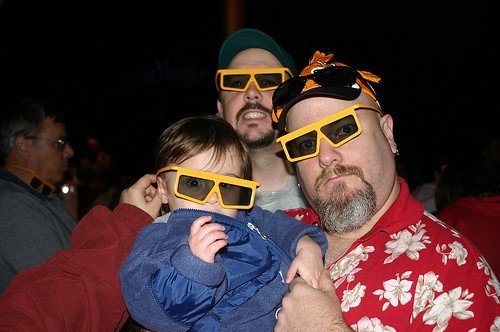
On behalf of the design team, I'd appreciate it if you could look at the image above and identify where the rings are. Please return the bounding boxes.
[274,307,281,319]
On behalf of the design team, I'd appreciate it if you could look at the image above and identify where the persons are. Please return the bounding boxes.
[410,139,500,281]
[0,174,165,332]
[0,101,80,296]
[270,50,500,332]
[77,136,128,210]
[214,30,308,213]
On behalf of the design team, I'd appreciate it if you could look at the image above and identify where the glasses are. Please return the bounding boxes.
[155,166,262,210]
[275,103,384,164]
[25,136,66,151]
[214,68,294,92]
[271,66,383,110]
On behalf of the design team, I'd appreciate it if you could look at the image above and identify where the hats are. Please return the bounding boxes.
[216,28,296,76]
[271,51,385,131]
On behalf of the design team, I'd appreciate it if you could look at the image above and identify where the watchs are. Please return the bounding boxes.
[119,116,328,332]
